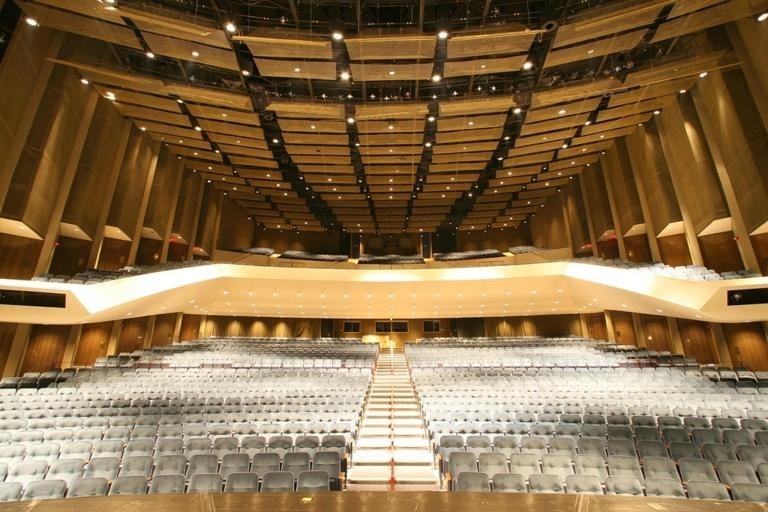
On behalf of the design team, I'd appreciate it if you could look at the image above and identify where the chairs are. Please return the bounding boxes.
[30,260,214,285]
[555,256,761,281]
